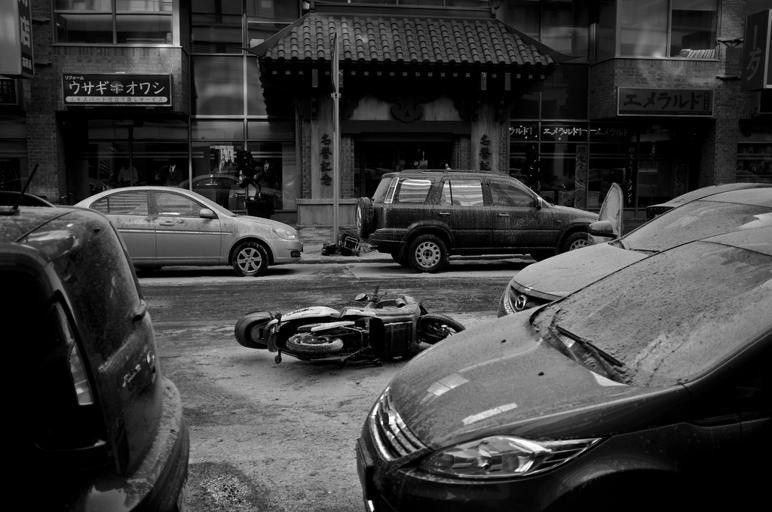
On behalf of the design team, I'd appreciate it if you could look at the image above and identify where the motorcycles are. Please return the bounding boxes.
[234,282,468,369]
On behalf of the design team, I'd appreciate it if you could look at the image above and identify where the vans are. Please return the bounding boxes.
[0,158,192,510]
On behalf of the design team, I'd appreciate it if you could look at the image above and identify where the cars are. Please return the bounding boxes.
[495,180,772,317]
[176,173,299,215]
[73,184,304,278]
[349,225,772,512]
[643,180,772,225]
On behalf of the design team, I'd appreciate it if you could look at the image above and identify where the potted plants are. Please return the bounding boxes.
[232,141,275,219]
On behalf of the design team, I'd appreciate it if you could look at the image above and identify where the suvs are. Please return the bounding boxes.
[353,161,601,274]
